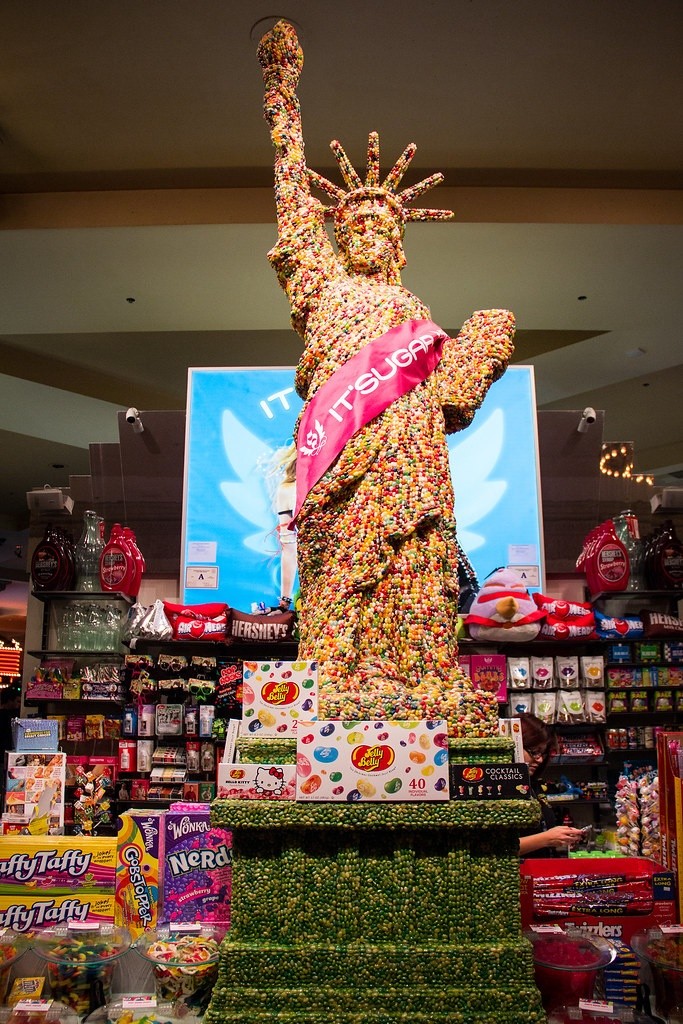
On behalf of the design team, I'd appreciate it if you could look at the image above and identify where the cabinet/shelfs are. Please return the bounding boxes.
[118,639,606,831]
[24,591,135,836]
[607,638,682,824]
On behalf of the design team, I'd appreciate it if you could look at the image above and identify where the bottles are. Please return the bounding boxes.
[575,519,629,590]
[31,523,75,591]
[59,603,122,651]
[623,509,641,590]
[99,523,144,597]
[643,520,683,590]
[75,511,103,592]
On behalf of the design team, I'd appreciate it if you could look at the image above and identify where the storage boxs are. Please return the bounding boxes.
[0,894,114,936]
[115,807,168,944]
[162,813,232,924]
[10,718,57,753]
[0,835,116,894]
[519,857,676,948]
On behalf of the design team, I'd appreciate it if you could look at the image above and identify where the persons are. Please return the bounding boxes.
[257,18,516,738]
[250,440,298,616]
[184,785,212,802]
[119,783,145,800]
[511,712,586,858]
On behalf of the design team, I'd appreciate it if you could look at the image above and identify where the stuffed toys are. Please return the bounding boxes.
[467,569,541,642]
